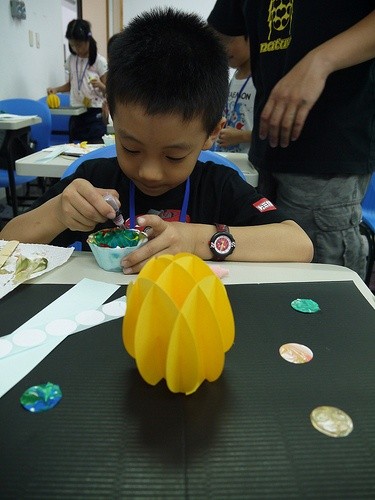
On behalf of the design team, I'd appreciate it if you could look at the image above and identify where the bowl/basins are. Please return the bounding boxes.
[86,228,148,272]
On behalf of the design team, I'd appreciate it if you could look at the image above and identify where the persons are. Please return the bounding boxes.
[47,19,107,144]
[208,27,257,153]
[205,0,375,282]
[0,6,314,274]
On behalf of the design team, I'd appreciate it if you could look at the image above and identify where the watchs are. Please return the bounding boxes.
[209,223,236,262]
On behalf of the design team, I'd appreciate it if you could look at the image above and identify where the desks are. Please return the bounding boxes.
[22,251,374,309]
[16,143,259,253]
[0,113,43,217]
[47,106,88,116]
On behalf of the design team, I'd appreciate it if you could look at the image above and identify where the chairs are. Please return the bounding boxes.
[39,93,71,147]
[359,172,375,286]
[0,97,51,211]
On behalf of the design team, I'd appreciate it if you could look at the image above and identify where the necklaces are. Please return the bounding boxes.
[224,67,252,129]
[130,175,190,228]
[76,54,88,96]
